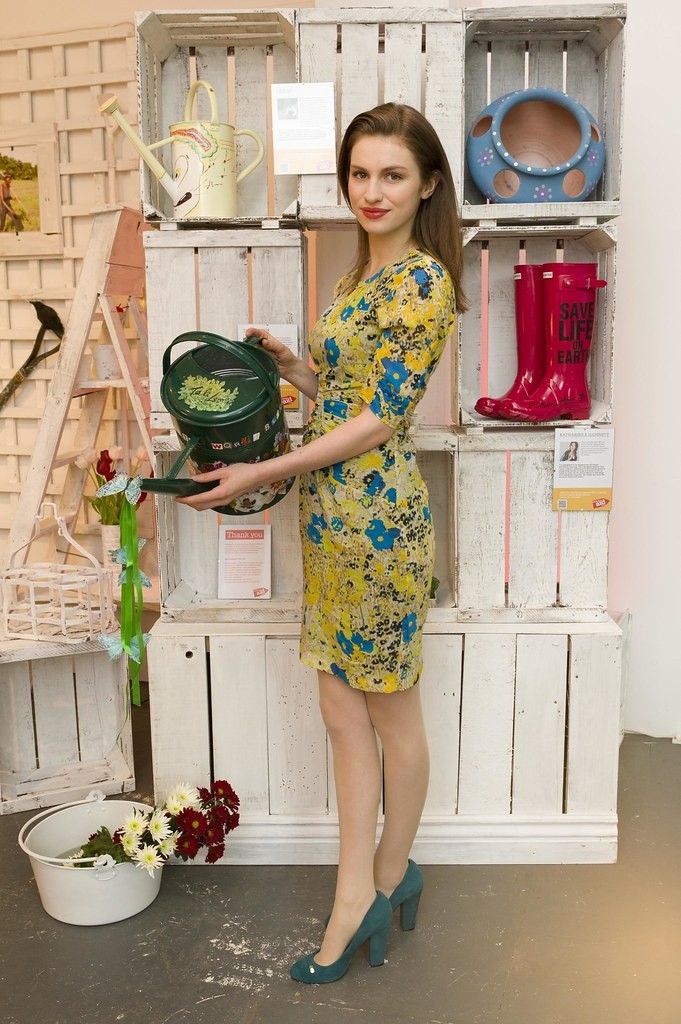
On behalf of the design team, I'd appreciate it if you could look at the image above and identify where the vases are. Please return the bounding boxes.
[90,344,122,379]
[101,525,121,591]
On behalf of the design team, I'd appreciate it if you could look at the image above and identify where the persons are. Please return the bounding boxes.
[176,103,469,986]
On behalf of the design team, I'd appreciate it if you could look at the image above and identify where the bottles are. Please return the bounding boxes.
[466,87,607,203]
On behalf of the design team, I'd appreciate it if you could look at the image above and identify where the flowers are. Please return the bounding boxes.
[63,779,241,879]
[74,446,155,525]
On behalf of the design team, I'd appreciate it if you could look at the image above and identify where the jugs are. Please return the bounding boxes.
[130,330,296,516]
[98,81,265,218]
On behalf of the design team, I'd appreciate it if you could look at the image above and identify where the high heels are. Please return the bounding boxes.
[326,859,423,932]
[290,890,393,984]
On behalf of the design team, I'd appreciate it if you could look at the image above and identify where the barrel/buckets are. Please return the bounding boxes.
[16,788,162,926]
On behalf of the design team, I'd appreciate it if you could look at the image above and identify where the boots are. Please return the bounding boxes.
[474,263,607,422]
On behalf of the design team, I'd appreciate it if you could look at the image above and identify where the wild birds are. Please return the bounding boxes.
[29,301,65,335]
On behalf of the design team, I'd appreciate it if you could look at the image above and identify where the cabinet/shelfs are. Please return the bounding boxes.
[139,2,630,625]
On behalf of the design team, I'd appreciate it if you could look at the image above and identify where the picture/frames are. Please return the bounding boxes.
[0,122,66,257]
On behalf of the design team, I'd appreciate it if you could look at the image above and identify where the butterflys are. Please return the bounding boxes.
[95,472,143,506]
